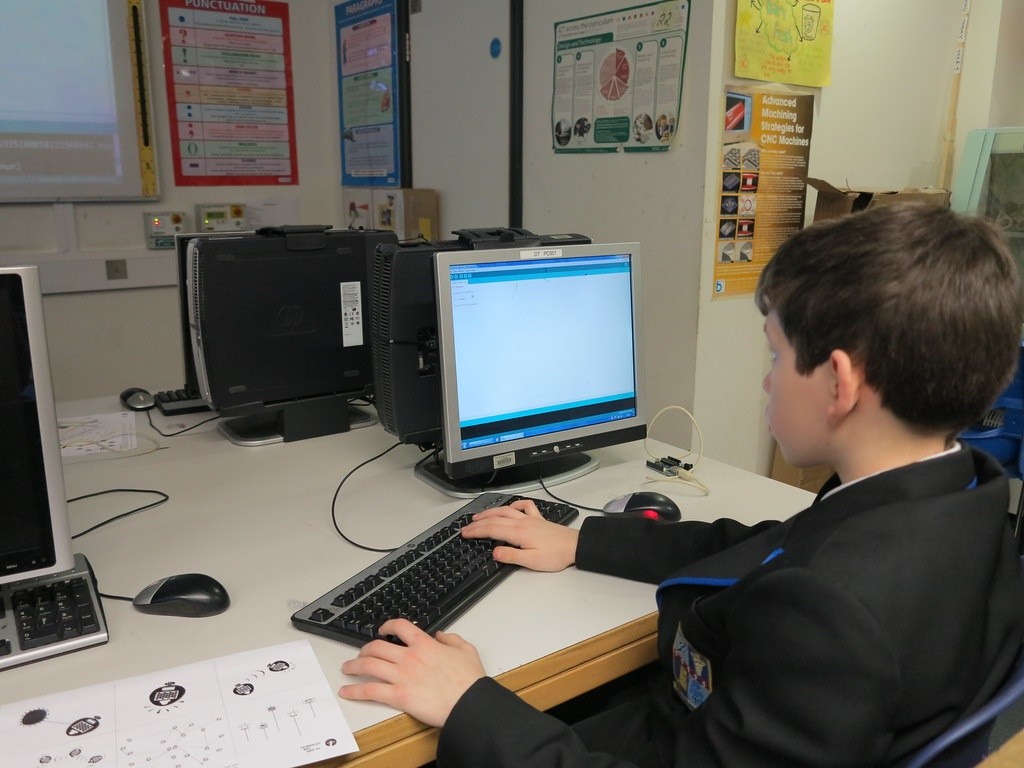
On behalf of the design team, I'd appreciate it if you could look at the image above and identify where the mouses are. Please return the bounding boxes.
[602,491,682,522]
[119,388,155,411]
[133,574,230,617]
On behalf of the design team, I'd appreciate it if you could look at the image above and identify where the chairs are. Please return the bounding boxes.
[906,668,1024,768]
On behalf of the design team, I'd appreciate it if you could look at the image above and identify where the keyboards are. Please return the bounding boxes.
[0,552,110,668]
[153,389,213,416]
[290,493,578,647]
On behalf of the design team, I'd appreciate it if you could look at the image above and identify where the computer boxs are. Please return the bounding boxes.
[188,227,398,412]
[370,227,591,445]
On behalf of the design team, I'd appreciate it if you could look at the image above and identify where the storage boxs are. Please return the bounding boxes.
[771,174,952,494]
[342,187,440,243]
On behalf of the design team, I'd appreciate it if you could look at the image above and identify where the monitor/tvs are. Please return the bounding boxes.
[0,265,75,586]
[176,230,255,393]
[415,240,645,497]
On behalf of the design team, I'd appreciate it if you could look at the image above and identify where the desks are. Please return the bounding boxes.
[0,388,818,768]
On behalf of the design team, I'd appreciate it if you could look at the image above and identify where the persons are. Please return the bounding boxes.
[655,114,675,142]
[635,114,646,143]
[338,202,1024,768]
